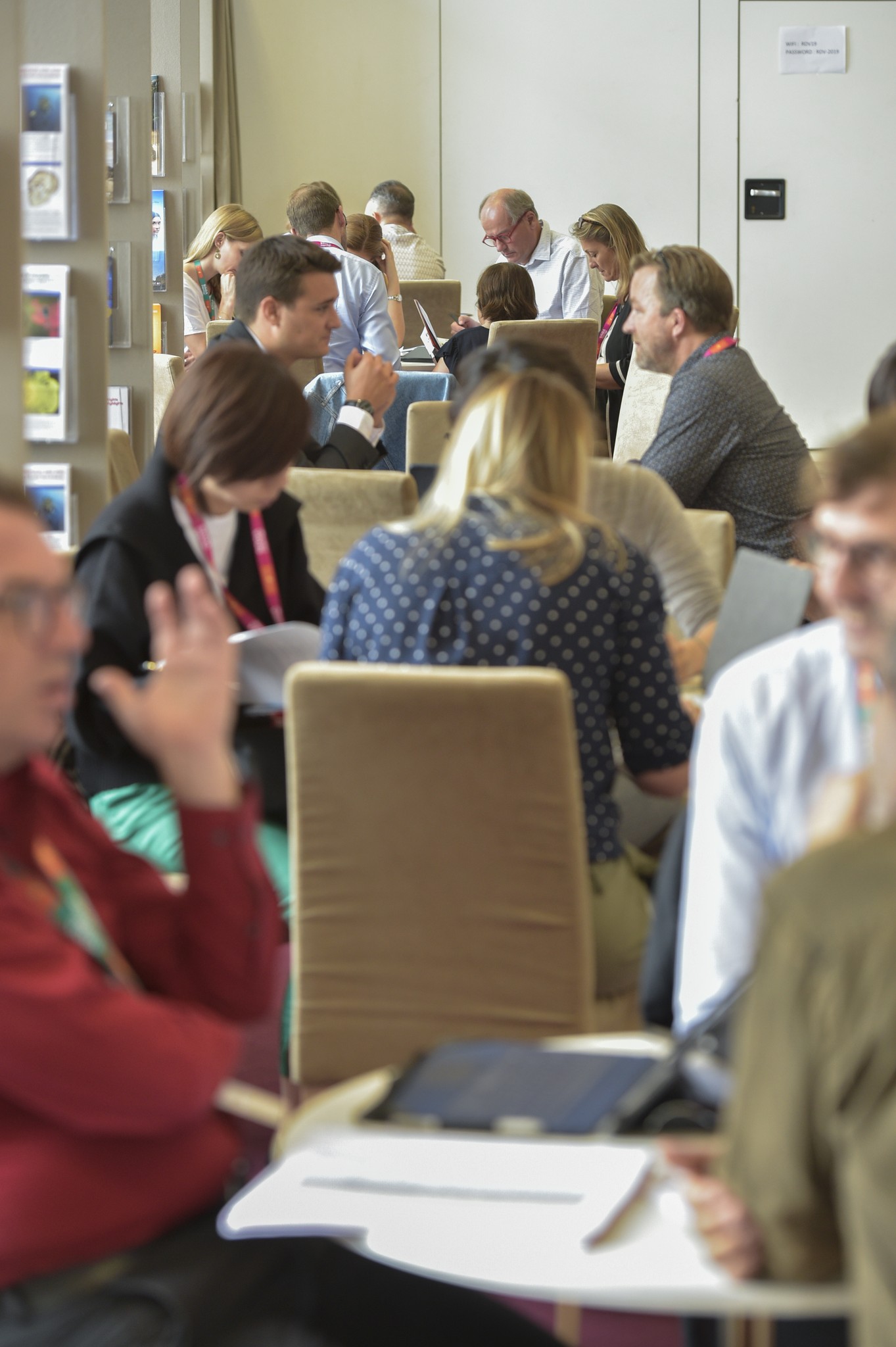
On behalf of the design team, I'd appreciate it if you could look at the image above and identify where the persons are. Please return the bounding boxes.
[669,404,895,1101]
[182,204,265,365]
[342,213,406,348]
[286,181,404,372]
[448,188,604,332]
[3,471,571,1346]
[321,364,698,999]
[429,262,539,393]
[658,605,896,1347]
[363,179,448,280]
[149,233,400,468]
[620,245,814,565]
[568,204,654,460]
[152,211,161,239]
[51,336,328,918]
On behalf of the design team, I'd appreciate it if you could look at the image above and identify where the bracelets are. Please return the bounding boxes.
[217,311,232,320]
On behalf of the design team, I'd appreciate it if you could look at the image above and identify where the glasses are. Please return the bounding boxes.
[0,575,89,648]
[578,217,603,229]
[482,208,536,247]
[793,522,895,588]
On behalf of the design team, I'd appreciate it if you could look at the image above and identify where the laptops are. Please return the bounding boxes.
[349,964,758,1141]
[678,544,814,697]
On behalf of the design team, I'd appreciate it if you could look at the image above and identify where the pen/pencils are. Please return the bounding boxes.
[450,314,465,327]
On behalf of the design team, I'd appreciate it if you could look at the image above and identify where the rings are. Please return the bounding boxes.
[184,359,187,364]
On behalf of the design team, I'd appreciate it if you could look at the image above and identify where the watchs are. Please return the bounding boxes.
[387,294,404,302]
[343,398,374,416]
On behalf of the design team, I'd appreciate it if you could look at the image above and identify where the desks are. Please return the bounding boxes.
[263,1022,854,1345]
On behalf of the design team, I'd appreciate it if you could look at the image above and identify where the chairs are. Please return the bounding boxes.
[396,279,463,352]
[103,426,144,504]
[203,318,325,394]
[682,506,735,596]
[280,656,652,1096]
[407,403,455,469]
[487,318,600,410]
[152,353,187,445]
[279,466,423,595]
